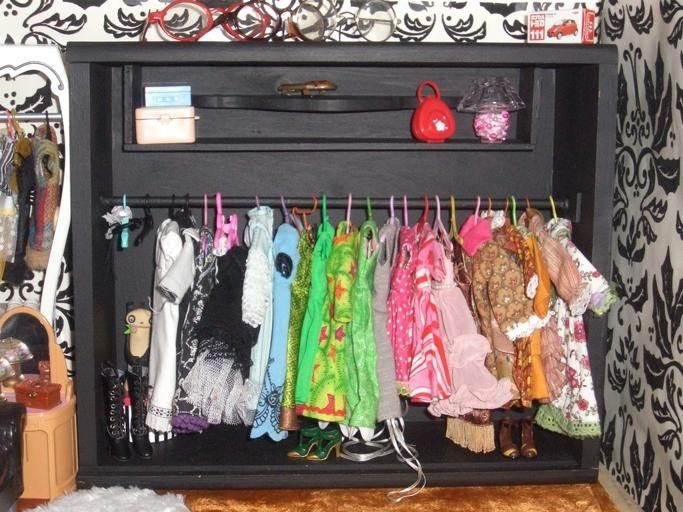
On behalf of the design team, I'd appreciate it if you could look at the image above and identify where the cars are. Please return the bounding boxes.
[548,19,579,40]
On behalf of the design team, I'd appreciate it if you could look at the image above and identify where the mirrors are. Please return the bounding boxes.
[0,308,75,405]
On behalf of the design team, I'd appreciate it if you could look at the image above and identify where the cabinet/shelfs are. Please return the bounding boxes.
[64,43,615,489]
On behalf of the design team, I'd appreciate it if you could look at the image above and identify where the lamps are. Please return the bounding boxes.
[458,77,527,144]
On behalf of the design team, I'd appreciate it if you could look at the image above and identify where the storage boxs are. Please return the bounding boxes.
[525,11,595,43]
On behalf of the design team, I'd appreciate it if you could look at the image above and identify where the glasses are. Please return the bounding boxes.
[139,0,401,44]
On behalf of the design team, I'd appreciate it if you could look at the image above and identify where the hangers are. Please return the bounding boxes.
[116,192,558,258]
[0,112,59,139]
